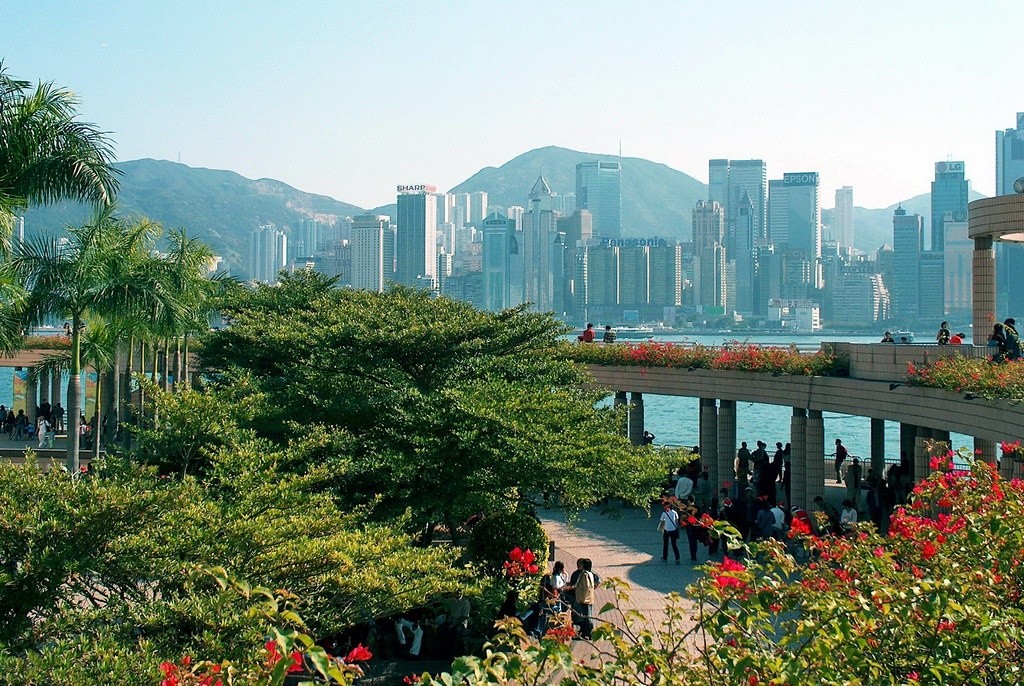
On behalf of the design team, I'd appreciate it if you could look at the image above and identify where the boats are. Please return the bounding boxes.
[891,330,914,341]
[593,327,654,339]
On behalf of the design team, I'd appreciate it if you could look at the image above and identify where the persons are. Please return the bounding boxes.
[643,431,655,445]
[831,439,847,483]
[550,559,601,640]
[319,591,470,661]
[844,458,908,533]
[80,414,107,449]
[603,325,617,343]
[0,399,64,449]
[578,323,595,343]
[987,318,1020,362]
[660,441,857,564]
[64,322,72,338]
[937,321,965,345]
[881,332,894,342]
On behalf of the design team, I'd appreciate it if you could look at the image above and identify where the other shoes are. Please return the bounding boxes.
[661,558,667,562]
[676,560,680,564]
[676,529,679,539]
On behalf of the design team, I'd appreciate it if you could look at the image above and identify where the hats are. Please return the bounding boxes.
[724,499,732,508]
[779,501,785,508]
